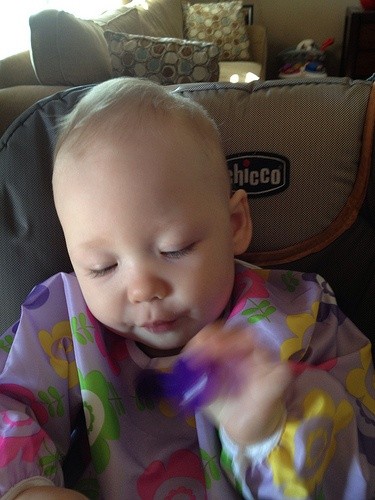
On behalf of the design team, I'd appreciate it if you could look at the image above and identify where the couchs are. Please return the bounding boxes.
[0,0,266,131]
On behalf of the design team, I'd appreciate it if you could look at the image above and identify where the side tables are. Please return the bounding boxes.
[338,6,375,80]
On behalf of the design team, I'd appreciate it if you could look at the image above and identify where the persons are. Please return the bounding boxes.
[0,76,375,500]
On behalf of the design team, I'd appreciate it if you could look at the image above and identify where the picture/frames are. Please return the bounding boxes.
[244,4,256,24]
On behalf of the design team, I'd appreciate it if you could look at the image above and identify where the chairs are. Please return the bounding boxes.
[0,79,375,336]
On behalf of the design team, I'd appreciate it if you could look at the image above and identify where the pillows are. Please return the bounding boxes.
[179,1,249,60]
[28,8,105,85]
[105,31,219,86]
